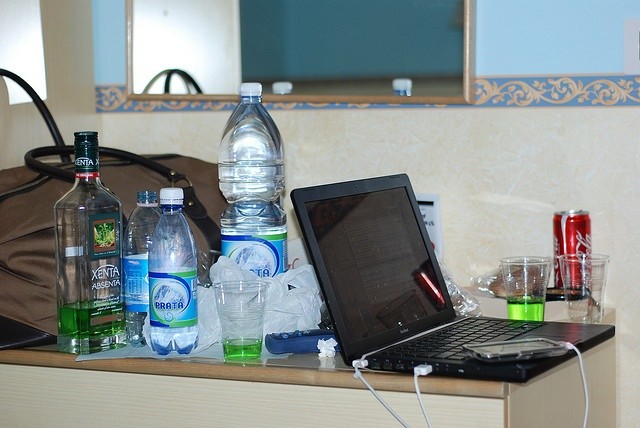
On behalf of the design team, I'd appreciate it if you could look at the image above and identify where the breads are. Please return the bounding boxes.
[489,259,550,300]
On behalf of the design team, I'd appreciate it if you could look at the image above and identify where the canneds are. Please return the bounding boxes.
[411,258,451,312]
[553,210,592,291]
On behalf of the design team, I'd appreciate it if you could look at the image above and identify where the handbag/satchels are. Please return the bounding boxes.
[1,68,228,349]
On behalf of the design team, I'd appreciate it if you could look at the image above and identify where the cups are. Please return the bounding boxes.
[501,255,551,321]
[213,280,272,362]
[558,254,609,324]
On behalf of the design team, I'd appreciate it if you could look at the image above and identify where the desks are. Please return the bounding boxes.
[1,307,616,428]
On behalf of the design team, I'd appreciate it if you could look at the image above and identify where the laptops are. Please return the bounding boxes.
[289,173,616,384]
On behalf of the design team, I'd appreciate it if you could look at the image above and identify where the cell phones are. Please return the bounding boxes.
[461,337,571,362]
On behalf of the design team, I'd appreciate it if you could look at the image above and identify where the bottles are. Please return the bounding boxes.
[148,187,198,356]
[122,190,161,349]
[218,83,288,278]
[271,81,293,97]
[54,132,123,355]
[392,78,412,97]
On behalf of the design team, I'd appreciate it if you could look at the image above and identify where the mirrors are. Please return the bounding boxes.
[125,0,475,104]
